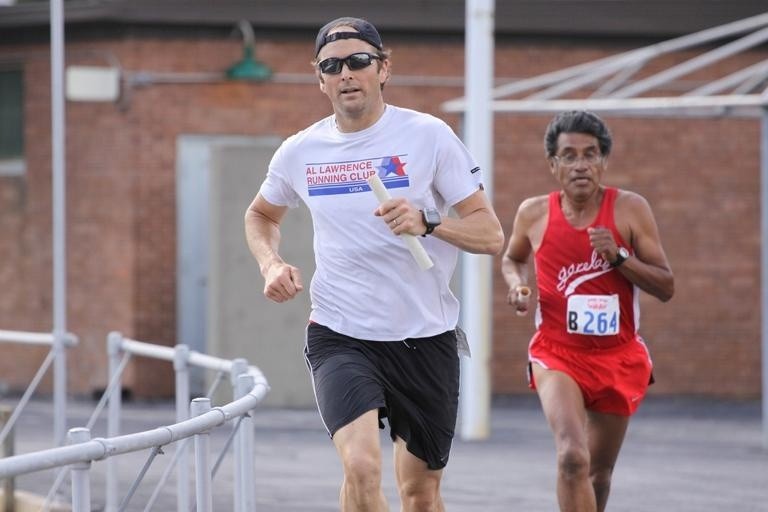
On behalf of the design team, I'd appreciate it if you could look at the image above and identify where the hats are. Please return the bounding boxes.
[314,17,383,57]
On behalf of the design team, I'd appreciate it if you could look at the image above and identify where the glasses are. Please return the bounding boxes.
[317,52,380,74]
[549,150,605,168]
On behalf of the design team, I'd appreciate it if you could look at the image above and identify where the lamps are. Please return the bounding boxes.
[225,18,276,84]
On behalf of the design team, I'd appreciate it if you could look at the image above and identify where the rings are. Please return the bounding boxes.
[393,219,400,225]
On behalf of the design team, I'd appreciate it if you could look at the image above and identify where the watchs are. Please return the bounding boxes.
[609,246,630,270]
[418,207,442,238]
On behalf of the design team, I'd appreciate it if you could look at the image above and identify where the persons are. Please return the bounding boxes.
[501,111,676,512]
[244,16,505,512]
